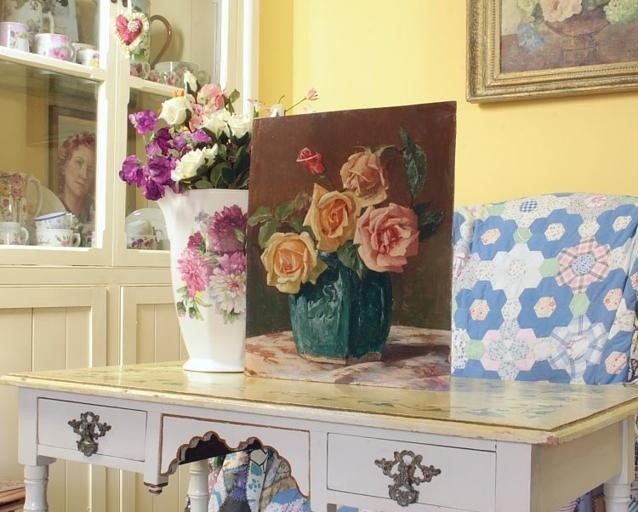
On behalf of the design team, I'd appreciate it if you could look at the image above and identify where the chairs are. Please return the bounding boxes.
[185,193,638,512]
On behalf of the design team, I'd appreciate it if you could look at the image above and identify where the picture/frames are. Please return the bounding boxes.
[465,2,637,104]
[49,106,137,246]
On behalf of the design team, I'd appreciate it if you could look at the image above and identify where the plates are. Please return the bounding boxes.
[125,208,170,249]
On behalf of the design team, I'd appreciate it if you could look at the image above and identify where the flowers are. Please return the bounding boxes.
[120,71,318,197]
[176,206,248,322]
[246,129,448,290]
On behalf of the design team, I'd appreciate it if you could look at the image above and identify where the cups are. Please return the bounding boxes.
[71,42,97,51]
[126,219,163,249]
[36,228,80,246]
[154,61,209,88]
[33,33,75,61]
[76,48,99,66]
[0,22,29,51]
[34,212,72,229]
[0,223,29,245]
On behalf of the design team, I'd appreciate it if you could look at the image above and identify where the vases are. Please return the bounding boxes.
[288,253,392,362]
[154,190,248,373]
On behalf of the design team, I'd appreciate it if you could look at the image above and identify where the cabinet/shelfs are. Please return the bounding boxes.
[0,0,226,509]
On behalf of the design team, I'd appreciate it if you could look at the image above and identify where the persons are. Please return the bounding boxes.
[56,131,96,223]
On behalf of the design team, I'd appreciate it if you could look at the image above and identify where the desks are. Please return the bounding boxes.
[1,366,638,512]
